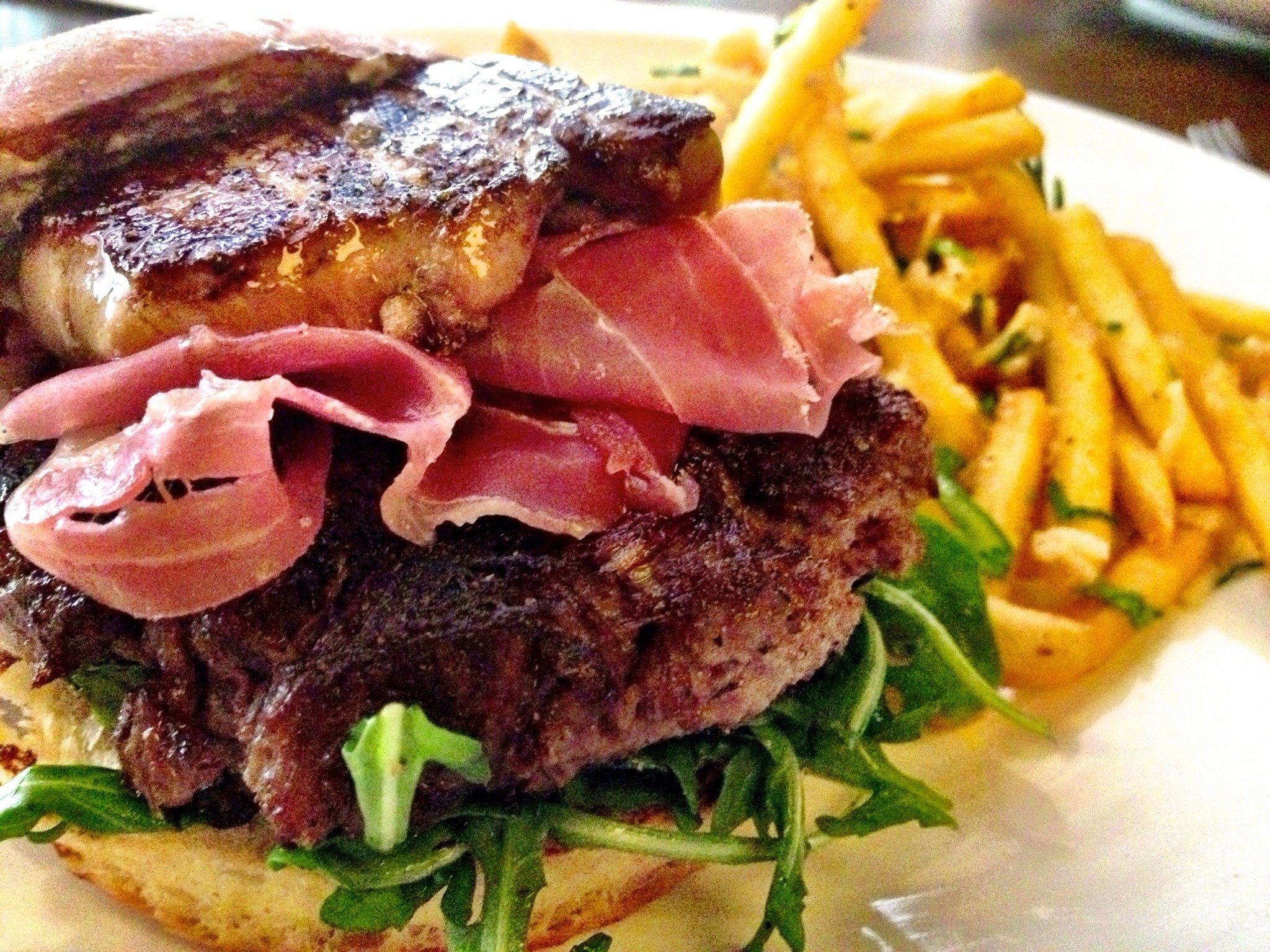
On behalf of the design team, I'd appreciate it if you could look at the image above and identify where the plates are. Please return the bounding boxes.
[0,32,1269,949]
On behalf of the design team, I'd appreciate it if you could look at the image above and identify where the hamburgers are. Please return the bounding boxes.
[0,0,1066,951]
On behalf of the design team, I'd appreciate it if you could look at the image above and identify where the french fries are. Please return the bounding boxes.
[457,0,1270,674]
